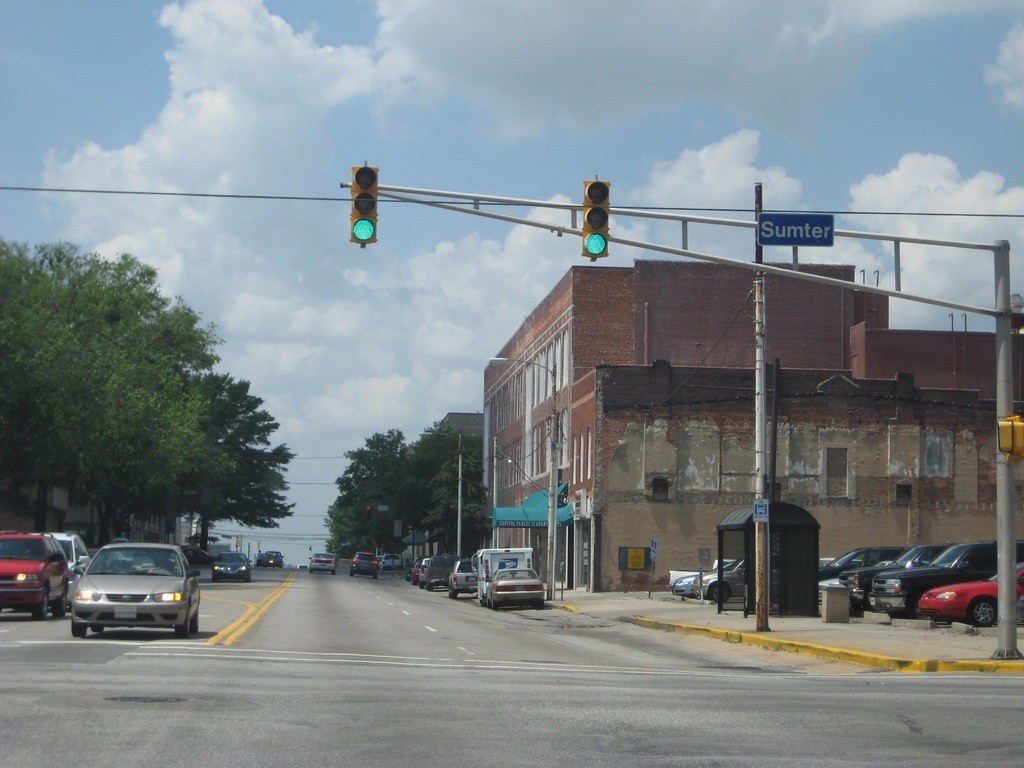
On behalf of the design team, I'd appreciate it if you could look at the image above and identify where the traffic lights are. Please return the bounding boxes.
[367,506,371,518]
[997,421,1024,453]
[309,547,311,553]
[349,166,379,243]
[582,180,610,259]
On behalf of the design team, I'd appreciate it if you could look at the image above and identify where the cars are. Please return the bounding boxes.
[692,558,746,602]
[405,556,450,592]
[211,551,252,584]
[258,551,284,568]
[310,553,336,574]
[818,544,952,611]
[671,562,734,599]
[484,568,545,611]
[917,563,1024,627]
[377,554,402,571]
[297,564,308,569]
[70,543,200,638]
[868,539,1024,619]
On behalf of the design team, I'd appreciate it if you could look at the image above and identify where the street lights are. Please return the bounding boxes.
[419,432,462,557]
[490,358,557,602]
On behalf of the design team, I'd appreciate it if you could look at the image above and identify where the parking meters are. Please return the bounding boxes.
[560,562,566,601]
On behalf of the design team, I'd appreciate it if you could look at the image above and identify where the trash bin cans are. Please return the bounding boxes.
[822,583,851,623]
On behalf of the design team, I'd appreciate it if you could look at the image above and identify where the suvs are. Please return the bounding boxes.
[350,553,378,578]
[0,528,93,620]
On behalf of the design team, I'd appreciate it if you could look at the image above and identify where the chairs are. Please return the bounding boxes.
[109,559,128,574]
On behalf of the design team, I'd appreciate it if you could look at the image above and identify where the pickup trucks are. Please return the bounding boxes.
[448,559,479,599]
[669,560,738,587]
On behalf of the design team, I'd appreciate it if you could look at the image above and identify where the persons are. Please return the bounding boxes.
[188,539,200,565]
[256,548,263,568]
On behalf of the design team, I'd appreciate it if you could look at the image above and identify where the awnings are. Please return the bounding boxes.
[492,482,574,529]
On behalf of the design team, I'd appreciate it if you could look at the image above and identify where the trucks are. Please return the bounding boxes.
[470,548,534,605]
